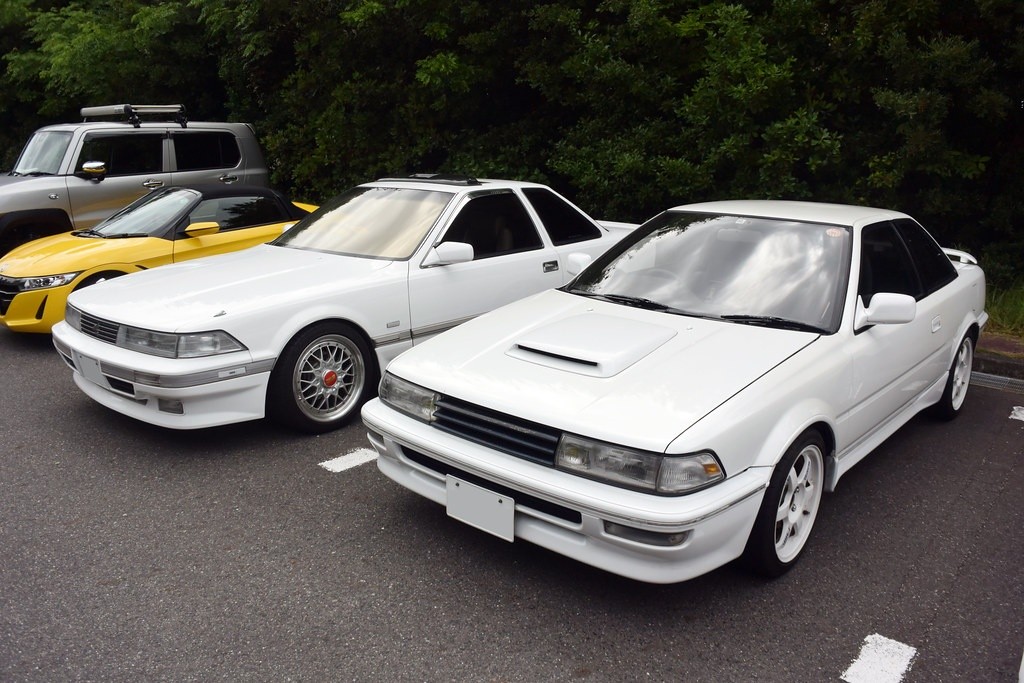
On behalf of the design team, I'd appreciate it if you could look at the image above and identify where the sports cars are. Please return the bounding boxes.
[0,184,334,336]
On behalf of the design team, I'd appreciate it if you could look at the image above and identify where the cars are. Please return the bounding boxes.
[49,174,653,434]
[359,197,989,587]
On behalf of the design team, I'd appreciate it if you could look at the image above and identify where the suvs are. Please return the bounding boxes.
[0,99,274,264]
